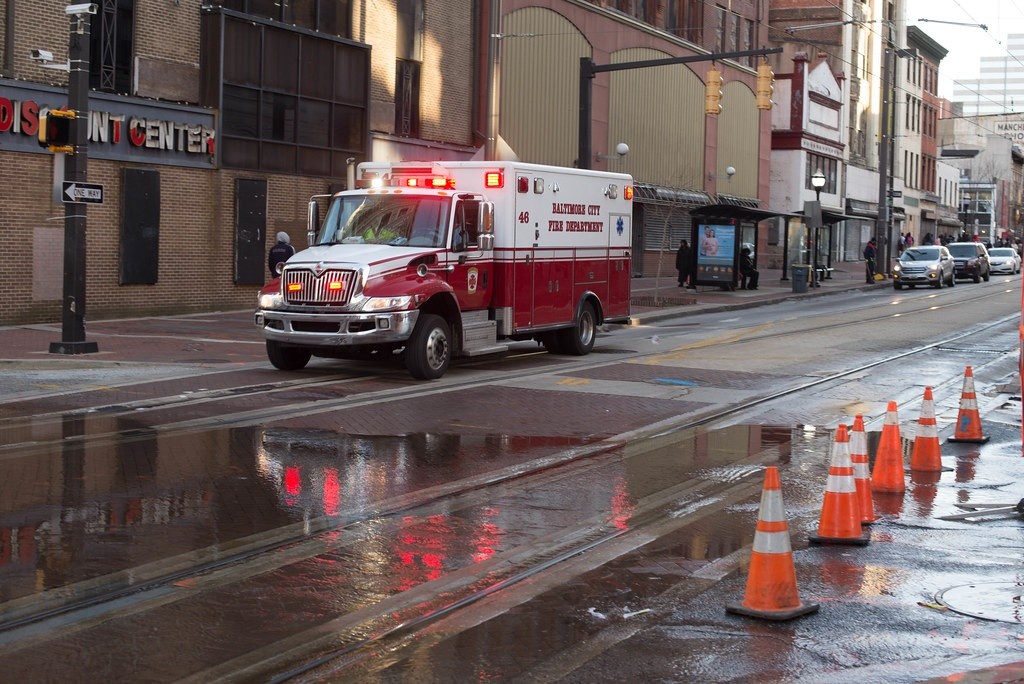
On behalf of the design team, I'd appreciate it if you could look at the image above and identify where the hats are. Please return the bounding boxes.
[277,232,290,243]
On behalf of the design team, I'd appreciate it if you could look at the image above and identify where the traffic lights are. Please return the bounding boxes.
[705,71,724,115]
[757,65,775,110]
[39,108,75,152]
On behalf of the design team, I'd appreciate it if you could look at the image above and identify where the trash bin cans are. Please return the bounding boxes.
[792,264,811,292]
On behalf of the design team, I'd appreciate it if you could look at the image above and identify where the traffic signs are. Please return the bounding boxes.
[61,181,107,204]
[886,191,902,198]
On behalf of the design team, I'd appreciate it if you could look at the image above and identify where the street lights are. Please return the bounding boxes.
[809,169,825,287]
[873,46,920,280]
[963,193,972,237]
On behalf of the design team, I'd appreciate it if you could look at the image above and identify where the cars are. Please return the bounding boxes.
[987,248,1022,275]
[892,246,958,287]
[743,241,755,266]
[945,242,991,282]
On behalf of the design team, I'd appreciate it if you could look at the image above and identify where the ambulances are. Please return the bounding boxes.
[253,160,636,382]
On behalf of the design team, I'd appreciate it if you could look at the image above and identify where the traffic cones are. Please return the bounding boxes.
[808,424,871,546]
[946,366,992,444]
[904,386,955,473]
[726,466,822,621]
[870,401,909,494]
[848,414,883,524]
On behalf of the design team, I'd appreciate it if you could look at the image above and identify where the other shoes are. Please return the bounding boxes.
[747,285,758,290]
[678,283,684,287]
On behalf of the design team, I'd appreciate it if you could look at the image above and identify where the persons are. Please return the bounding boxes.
[699,226,720,257]
[676,239,692,286]
[898,232,1023,258]
[740,248,759,290]
[363,216,400,239]
[439,215,470,247]
[864,238,877,284]
[268,231,296,280]
